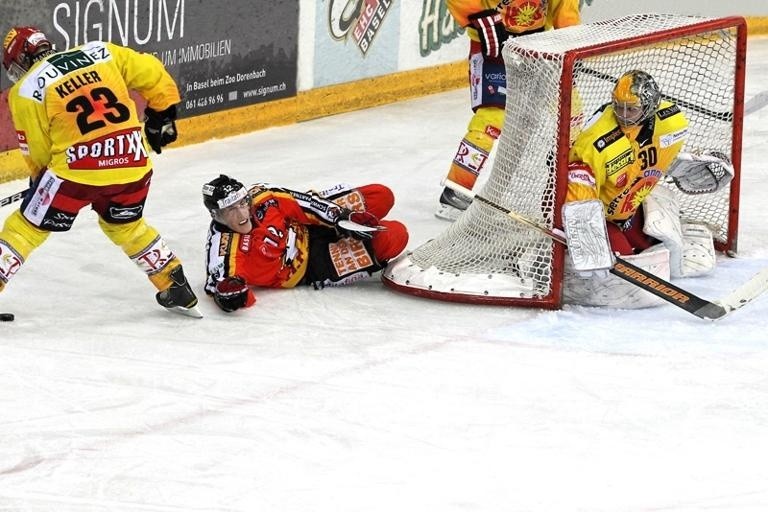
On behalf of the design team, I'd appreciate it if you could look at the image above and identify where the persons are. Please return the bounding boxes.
[432,0,583,224]
[203,172,411,312]
[0,25,202,323]
[563,69,735,310]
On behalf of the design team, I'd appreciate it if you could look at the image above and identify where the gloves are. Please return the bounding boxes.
[144,104,176,154]
[468,9,506,60]
[333,209,378,240]
[213,276,247,312]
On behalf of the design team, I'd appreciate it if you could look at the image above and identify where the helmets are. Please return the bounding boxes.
[202,175,248,213]
[610,69,660,129]
[2,25,51,84]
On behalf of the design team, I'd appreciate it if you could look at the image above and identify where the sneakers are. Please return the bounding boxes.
[155,265,197,309]
[439,186,472,210]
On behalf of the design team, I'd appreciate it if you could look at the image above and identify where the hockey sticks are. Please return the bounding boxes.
[445,177,755,322]
[505,42,767,122]
